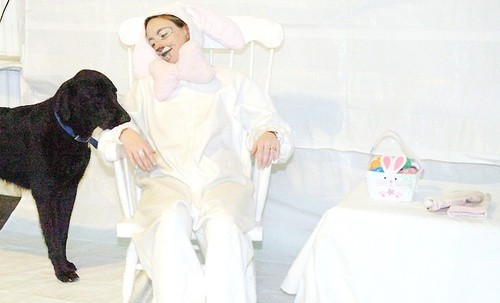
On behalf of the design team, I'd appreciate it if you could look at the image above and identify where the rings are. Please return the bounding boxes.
[269,147,277,151]
[139,150,144,158]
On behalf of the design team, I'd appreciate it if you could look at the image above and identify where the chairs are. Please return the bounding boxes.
[111,20,286,303]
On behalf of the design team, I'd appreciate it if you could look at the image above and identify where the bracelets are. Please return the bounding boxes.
[269,131,280,140]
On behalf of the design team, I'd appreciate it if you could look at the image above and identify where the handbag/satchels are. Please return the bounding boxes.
[364,131,423,203]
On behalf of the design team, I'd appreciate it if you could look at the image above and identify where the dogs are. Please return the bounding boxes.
[0,69,132,282]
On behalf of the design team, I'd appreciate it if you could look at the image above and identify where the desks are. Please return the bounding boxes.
[281,176,499,303]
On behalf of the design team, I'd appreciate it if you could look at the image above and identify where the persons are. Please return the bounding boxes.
[97,5,292,303]
